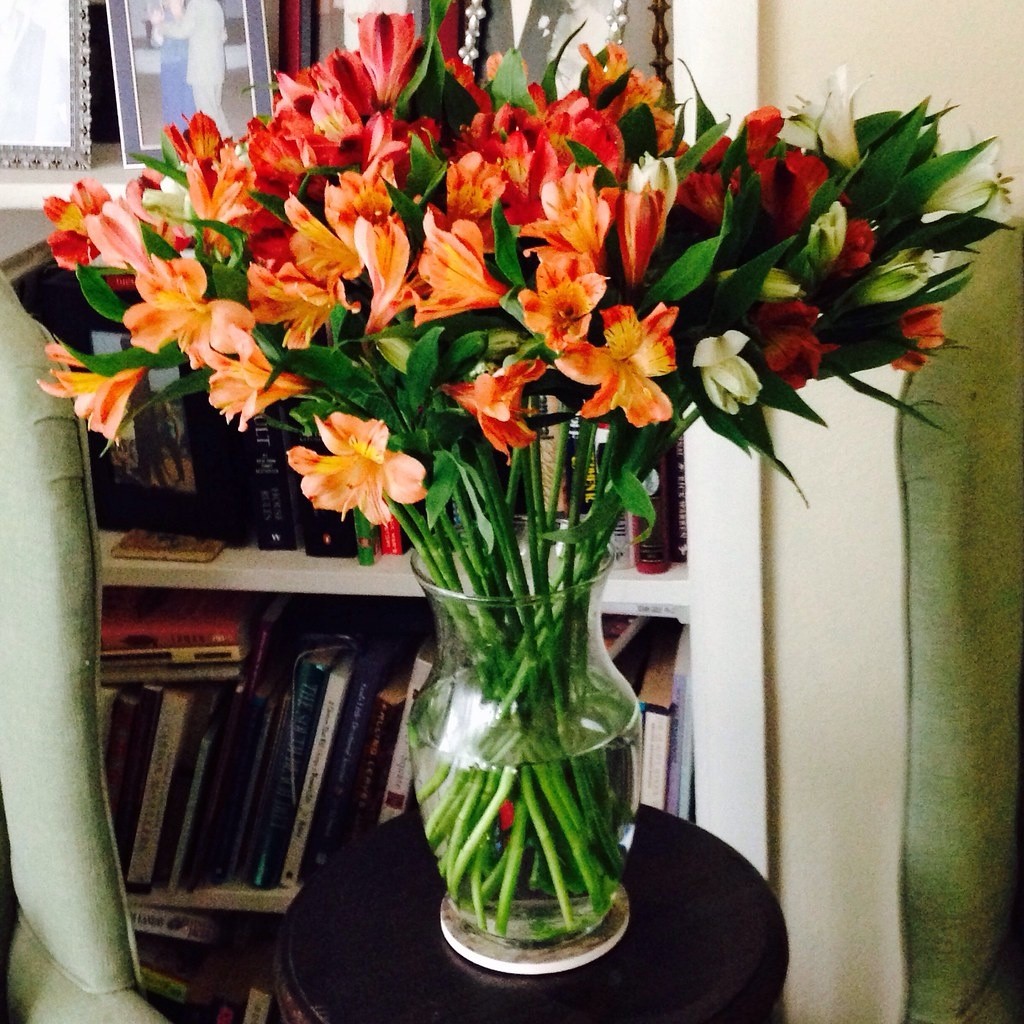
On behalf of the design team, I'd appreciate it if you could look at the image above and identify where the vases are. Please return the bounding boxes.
[407,514,642,974]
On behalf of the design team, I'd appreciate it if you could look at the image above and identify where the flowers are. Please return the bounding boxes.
[25,0,1017,935]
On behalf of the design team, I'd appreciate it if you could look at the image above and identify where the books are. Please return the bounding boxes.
[82,361,696,1024]
[96,602,694,1024]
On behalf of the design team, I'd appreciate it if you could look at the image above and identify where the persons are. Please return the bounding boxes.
[474,0,573,87]
[151,0,235,148]
[333,0,407,55]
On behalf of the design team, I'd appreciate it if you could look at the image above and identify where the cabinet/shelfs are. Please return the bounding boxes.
[0,1,768,1024]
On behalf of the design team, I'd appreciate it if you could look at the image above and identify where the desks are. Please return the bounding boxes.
[273,803,788,1024]
